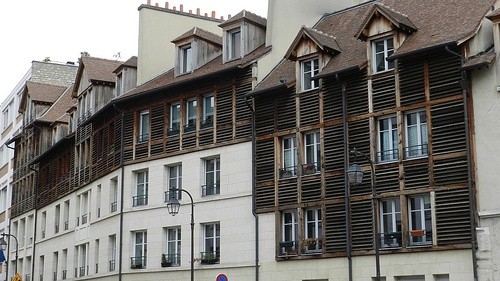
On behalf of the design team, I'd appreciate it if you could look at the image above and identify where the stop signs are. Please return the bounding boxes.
[216,274,228,281]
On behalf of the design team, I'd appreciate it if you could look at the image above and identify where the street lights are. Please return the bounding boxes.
[166,185,194,281]
[0,232,18,275]
[345,147,381,281]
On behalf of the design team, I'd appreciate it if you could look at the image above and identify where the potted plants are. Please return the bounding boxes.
[201,253,215,264]
[161,253,171,267]
